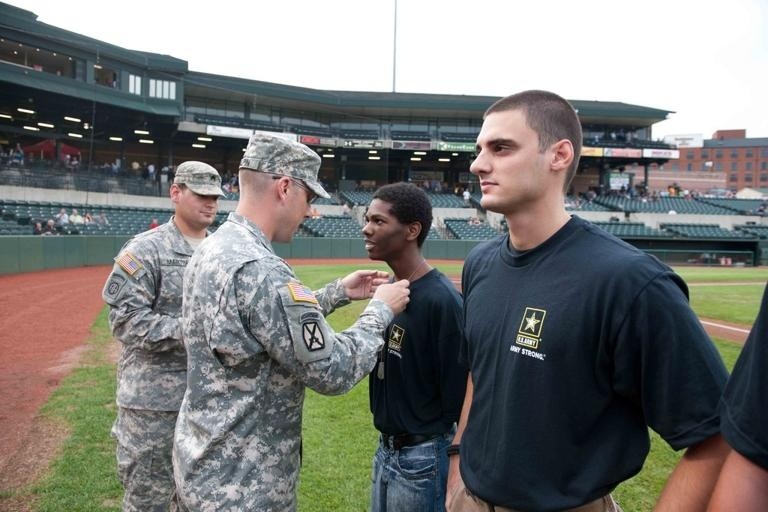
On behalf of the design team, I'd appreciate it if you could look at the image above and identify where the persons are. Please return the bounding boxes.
[171,135,412,512]
[444,89,730,512]
[361,182,463,512]
[706,286,768,512]
[102,160,225,512]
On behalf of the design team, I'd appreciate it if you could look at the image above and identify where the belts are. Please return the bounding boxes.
[381,432,440,450]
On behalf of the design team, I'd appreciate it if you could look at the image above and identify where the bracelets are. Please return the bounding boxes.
[446,444,462,457]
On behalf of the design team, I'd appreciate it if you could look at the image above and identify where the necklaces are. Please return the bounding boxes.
[377,258,427,379]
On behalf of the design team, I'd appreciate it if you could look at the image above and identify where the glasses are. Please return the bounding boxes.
[271,175,319,205]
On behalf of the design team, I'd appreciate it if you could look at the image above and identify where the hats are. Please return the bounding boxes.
[237,133,331,199]
[173,160,227,198]
